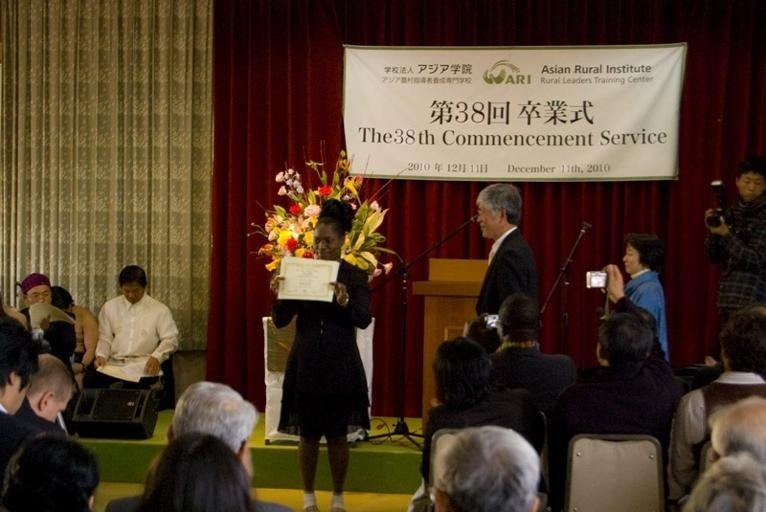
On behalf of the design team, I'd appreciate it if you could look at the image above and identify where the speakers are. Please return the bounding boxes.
[67,387,156,440]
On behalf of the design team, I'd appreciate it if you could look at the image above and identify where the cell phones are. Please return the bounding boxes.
[585,271,607,288]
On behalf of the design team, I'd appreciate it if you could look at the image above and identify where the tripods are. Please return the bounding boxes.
[359,217,475,451]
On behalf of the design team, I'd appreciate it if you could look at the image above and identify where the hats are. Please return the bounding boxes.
[21,272,51,292]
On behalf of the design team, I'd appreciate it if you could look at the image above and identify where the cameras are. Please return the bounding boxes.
[707,180,735,228]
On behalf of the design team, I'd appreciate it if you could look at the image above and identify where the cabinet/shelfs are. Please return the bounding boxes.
[414,257,491,438]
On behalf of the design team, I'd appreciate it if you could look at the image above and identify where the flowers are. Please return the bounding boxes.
[247,141,403,294]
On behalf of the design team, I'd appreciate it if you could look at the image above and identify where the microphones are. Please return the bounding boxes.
[580,219,592,235]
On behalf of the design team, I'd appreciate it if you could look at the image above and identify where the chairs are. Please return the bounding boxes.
[564,434,665,511]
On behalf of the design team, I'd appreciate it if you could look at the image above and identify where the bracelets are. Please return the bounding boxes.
[345,292,350,303]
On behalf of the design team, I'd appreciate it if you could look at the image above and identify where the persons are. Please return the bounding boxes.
[0,433,100,512]
[704,154,766,324]
[623,233,670,363]
[0,293,28,331]
[94,265,179,410]
[105,381,294,512]
[601,263,665,358]
[546,313,675,512]
[19,273,81,397]
[430,425,543,512]
[475,183,537,316]
[667,306,766,504]
[0,316,47,497]
[13,353,73,432]
[51,286,98,393]
[491,292,577,410]
[270,198,372,512]
[133,431,257,512]
[419,336,548,493]
[682,394,766,512]
